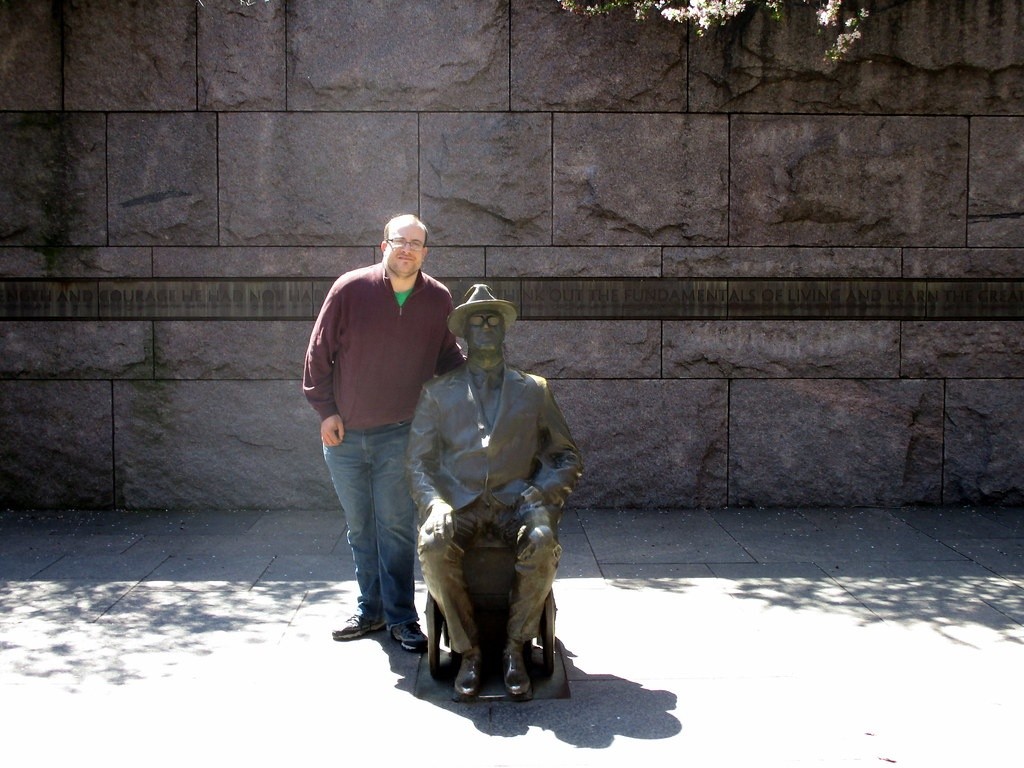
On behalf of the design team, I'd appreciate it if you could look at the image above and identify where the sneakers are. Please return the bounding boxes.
[389,621,428,653]
[332,612,387,641]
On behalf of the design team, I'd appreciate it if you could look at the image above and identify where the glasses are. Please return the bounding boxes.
[386,237,423,250]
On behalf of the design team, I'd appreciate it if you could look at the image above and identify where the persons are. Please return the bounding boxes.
[303,213,466,653]
[404,283,582,696]
